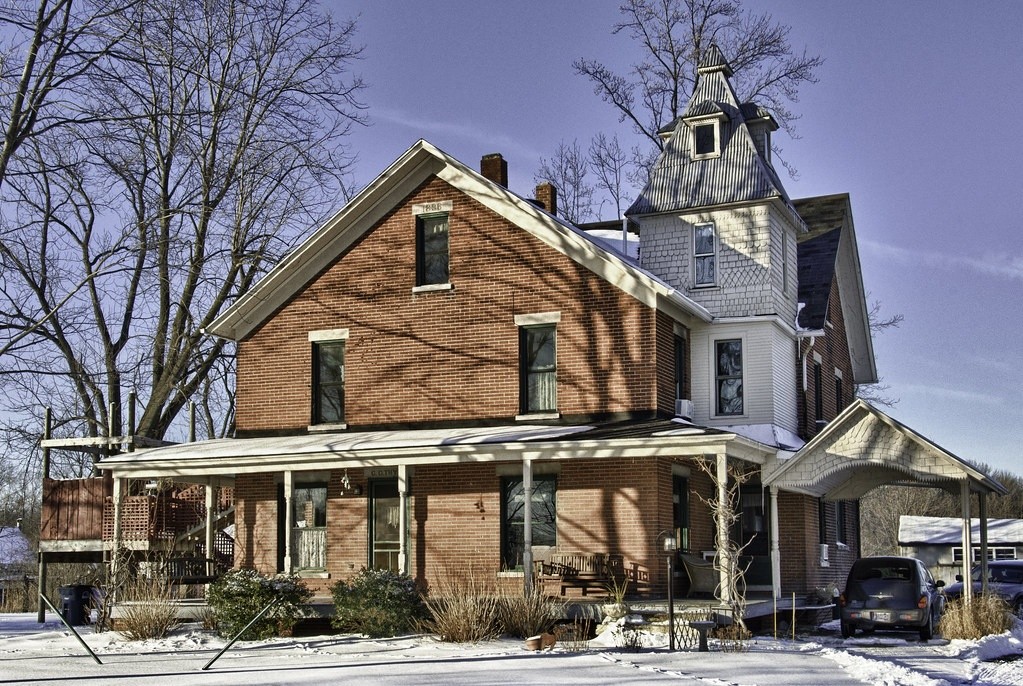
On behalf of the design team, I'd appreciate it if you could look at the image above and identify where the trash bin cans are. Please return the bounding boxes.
[832,598,841,620]
[59,583,95,626]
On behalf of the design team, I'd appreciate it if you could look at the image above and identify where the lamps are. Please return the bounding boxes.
[340,483,364,497]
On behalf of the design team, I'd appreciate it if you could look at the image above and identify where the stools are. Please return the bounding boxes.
[689,621,716,653]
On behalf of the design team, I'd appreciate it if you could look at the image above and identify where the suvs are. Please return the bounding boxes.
[839,556,946,640]
[941,560,1023,620]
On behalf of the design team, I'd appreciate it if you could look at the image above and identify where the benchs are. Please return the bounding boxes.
[535,550,630,600]
[158,556,218,597]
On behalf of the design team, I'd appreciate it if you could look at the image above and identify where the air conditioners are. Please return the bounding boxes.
[675,399,694,420]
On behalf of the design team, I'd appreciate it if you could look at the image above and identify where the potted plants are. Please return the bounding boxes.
[807,583,834,605]
[599,566,634,619]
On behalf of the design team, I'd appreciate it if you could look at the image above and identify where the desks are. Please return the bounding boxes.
[700,550,719,562]
